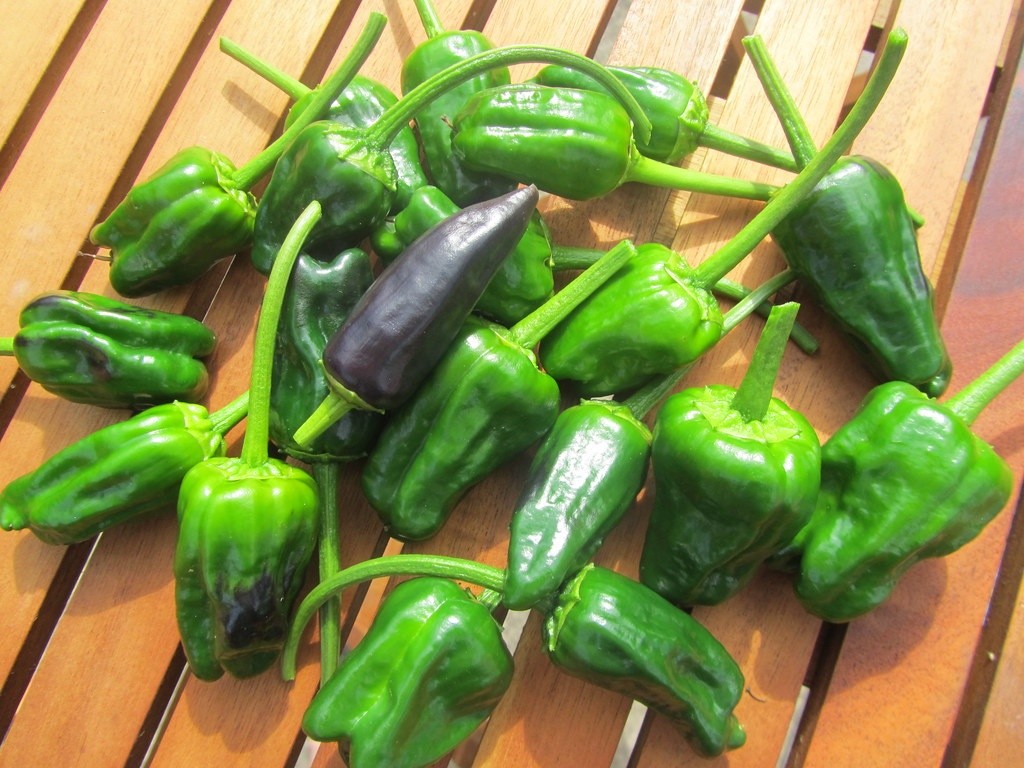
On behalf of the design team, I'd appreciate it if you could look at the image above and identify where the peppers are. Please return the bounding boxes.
[0,0,1024,768]
